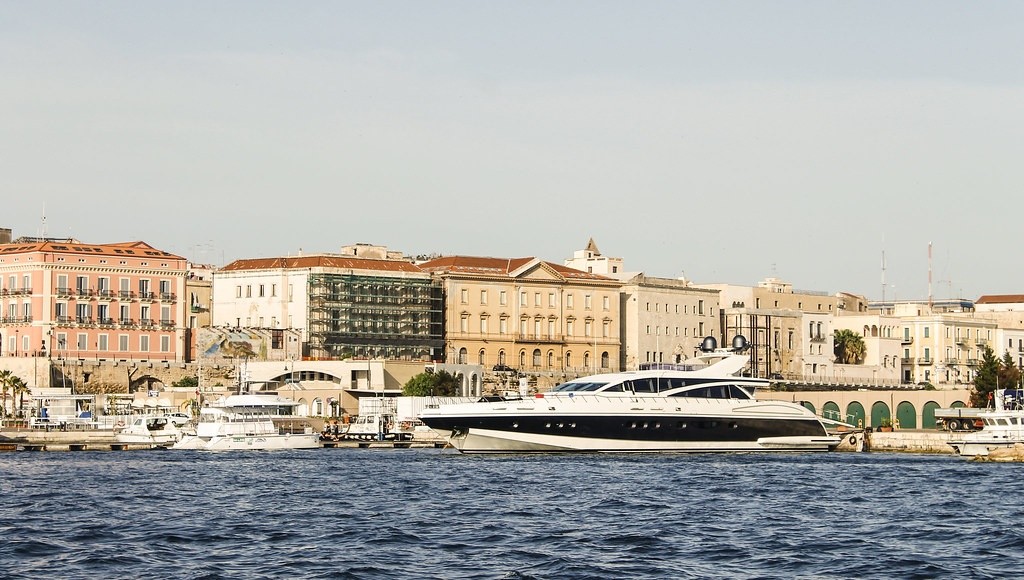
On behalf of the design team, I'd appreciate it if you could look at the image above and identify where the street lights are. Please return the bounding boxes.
[284,360,295,415]
[15,330,19,358]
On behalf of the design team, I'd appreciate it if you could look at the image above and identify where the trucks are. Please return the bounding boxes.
[934,389,1024,431]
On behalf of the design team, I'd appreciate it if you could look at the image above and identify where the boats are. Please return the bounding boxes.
[115,415,178,443]
[418,336,841,453]
[946,376,1024,460]
[167,406,323,451]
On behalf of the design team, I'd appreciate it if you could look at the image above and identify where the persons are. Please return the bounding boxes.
[942,417,947,431]
[988,392,993,409]
[153,419,161,428]
[334,422,340,441]
[326,422,331,435]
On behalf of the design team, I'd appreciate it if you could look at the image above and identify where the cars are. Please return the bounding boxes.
[164,413,192,427]
